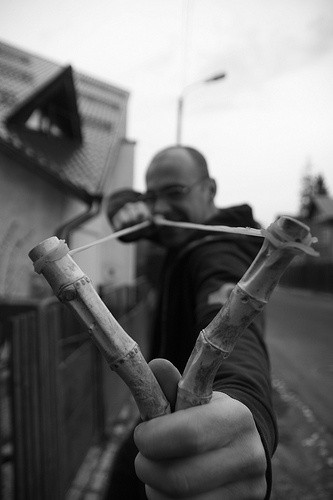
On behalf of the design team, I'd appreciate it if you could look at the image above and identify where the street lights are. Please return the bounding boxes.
[174,72,224,145]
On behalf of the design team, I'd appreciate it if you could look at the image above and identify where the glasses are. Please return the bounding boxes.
[144,174,208,207]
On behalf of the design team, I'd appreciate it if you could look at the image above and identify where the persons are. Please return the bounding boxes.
[105,143,281,500]
